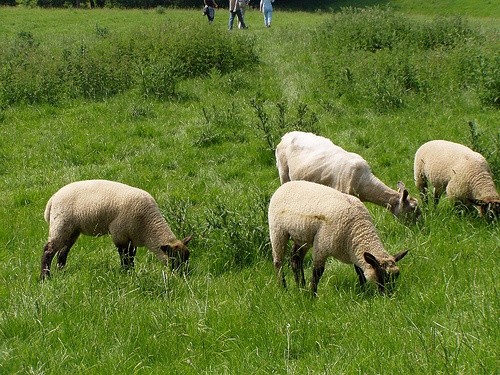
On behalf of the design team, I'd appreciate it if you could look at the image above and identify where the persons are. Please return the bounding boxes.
[228,0,250,30]
[204,0,218,23]
[260,0,274,27]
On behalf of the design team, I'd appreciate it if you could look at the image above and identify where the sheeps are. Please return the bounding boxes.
[411,138,500,222]
[266,179,412,299]
[39,179,195,286]
[274,129,428,228]
